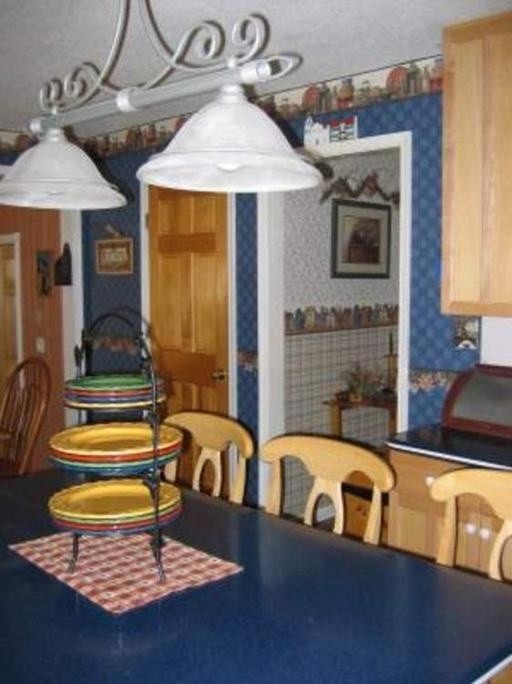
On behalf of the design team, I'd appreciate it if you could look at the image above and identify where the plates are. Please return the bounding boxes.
[45,371,185,542]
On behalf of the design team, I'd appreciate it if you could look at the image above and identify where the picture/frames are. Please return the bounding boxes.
[91,236,135,278]
[328,195,394,282]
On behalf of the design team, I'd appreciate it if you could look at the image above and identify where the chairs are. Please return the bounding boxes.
[160,410,257,505]
[0,357,53,478]
[425,465,512,582]
[256,434,396,548]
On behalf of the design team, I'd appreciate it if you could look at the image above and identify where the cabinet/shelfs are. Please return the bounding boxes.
[440,10,512,318]
[385,423,512,684]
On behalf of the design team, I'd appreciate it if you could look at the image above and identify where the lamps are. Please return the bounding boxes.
[0,0,325,212]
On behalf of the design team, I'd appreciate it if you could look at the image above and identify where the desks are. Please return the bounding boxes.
[320,389,397,547]
[1,467,512,684]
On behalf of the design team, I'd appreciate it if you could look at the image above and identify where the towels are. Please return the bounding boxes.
[8,530,246,615]
